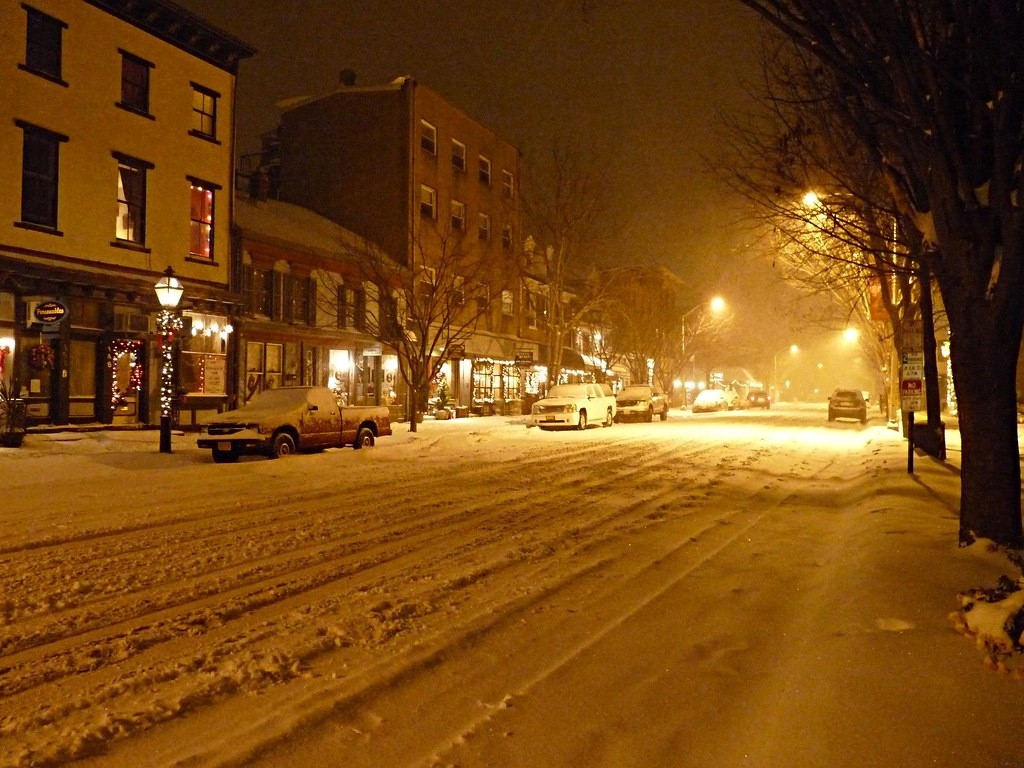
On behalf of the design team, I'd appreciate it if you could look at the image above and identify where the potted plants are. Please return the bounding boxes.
[0,376,30,448]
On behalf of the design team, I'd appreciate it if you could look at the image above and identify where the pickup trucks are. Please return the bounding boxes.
[196,386,393,461]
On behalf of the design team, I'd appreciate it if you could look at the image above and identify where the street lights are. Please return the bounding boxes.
[773,344,798,391]
[681,297,723,409]
[154,265,184,453]
[805,189,901,428]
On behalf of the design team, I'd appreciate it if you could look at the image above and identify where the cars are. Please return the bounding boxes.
[691,389,729,412]
[744,390,771,409]
[725,390,742,412]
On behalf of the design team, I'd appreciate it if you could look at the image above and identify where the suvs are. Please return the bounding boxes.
[827,389,870,424]
[615,384,669,423]
[531,383,617,429]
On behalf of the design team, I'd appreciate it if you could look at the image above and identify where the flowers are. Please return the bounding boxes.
[30,345,56,374]
